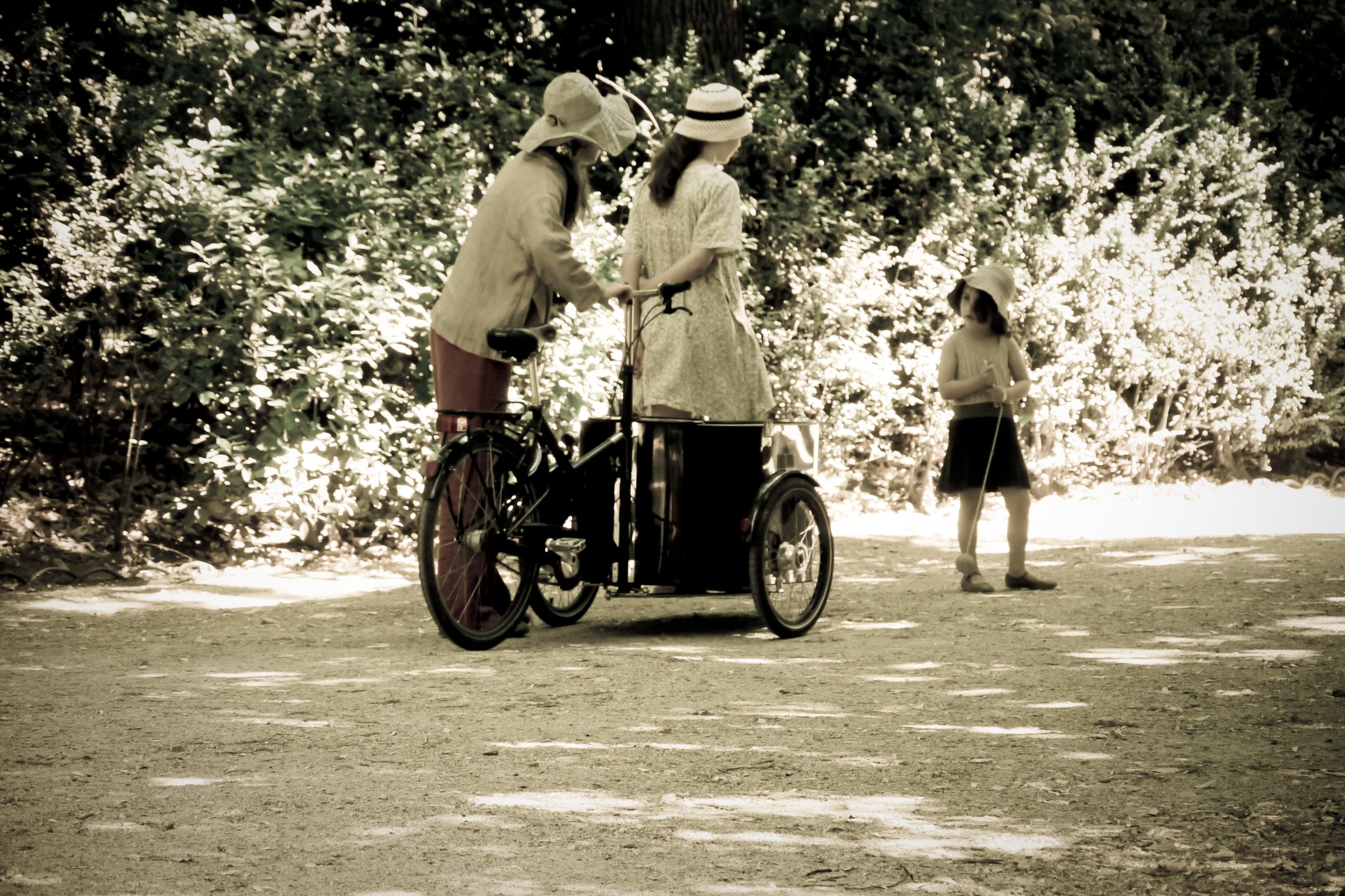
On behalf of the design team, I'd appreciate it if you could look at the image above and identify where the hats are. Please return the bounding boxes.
[947,263,1016,334]
[517,72,637,157]
[673,83,753,142]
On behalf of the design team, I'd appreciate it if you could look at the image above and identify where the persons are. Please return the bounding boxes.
[935,263,1061,595]
[619,82,779,425]
[424,71,639,644]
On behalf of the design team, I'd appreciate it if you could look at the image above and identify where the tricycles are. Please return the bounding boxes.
[414,277,836,651]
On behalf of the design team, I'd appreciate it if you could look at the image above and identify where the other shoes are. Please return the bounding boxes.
[960,570,995,592]
[521,614,530,624]
[1006,571,1057,590]
[509,621,528,636]
